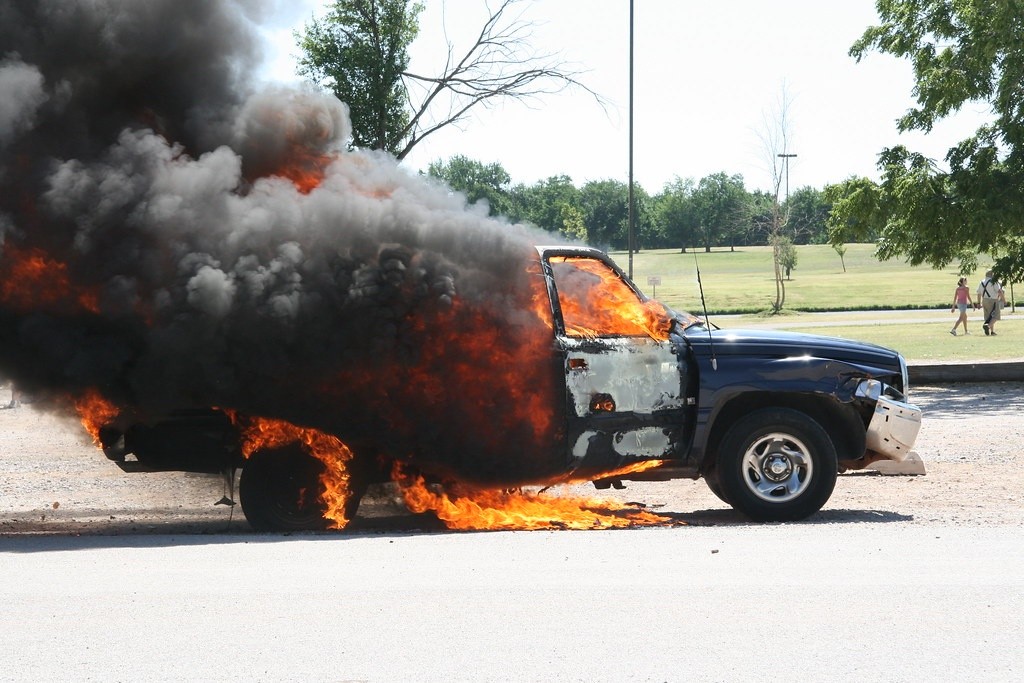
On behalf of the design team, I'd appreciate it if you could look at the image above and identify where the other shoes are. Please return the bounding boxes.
[983,324,990,335]
[965,332,972,335]
[950,329,957,336]
[991,332,997,336]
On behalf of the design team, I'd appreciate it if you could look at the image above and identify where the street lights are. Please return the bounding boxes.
[777,153,798,225]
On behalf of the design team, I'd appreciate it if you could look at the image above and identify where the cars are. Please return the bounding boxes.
[94,243,925,539]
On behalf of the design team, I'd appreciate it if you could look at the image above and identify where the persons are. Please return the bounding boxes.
[950,277,975,336]
[976,270,1006,335]
